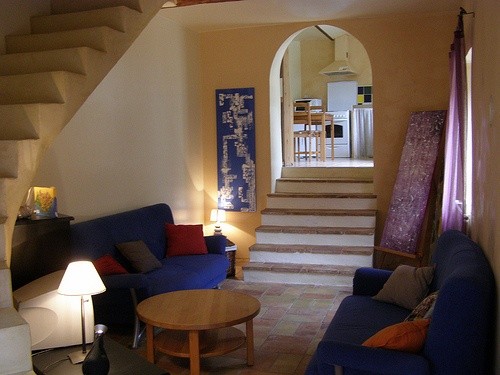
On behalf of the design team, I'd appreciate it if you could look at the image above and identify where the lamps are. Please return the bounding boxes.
[57,261,106,365]
[210,210,226,236]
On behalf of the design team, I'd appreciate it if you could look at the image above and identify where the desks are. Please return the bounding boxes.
[136,289,262,375]
[294,113,335,161]
[32,335,171,375]
[11,214,76,270]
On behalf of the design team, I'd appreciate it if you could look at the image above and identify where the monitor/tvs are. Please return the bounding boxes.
[12,269,94,350]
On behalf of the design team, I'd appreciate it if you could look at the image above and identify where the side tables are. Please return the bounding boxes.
[225,239,237,278]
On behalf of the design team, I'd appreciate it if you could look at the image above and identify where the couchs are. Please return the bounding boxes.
[304,229,500,375]
[38,203,231,348]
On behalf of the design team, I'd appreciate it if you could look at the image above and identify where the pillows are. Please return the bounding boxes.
[165,223,207,257]
[92,240,162,277]
[361,264,440,351]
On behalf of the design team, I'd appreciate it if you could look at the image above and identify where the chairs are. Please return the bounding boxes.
[294,103,326,162]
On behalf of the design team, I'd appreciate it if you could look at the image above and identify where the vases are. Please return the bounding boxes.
[82,324,110,375]
